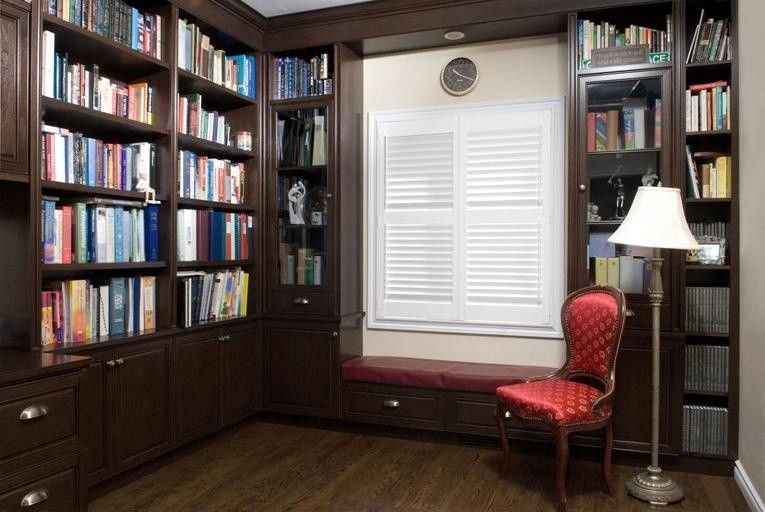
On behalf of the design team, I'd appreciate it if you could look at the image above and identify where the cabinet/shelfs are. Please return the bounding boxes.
[342,375,555,444]
[1,4,262,510]
[264,9,363,427]
[568,1,740,478]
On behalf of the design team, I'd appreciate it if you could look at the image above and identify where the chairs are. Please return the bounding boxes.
[494,286,628,511]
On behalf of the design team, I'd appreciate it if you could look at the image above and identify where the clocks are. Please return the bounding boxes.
[441,56,479,96]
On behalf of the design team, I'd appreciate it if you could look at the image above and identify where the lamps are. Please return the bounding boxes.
[608,185,701,505]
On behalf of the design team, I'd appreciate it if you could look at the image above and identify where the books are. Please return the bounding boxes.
[578,14,671,293]
[682,9,732,456]
[273,53,333,286]
[177,16,255,327]
[41,0,160,345]
